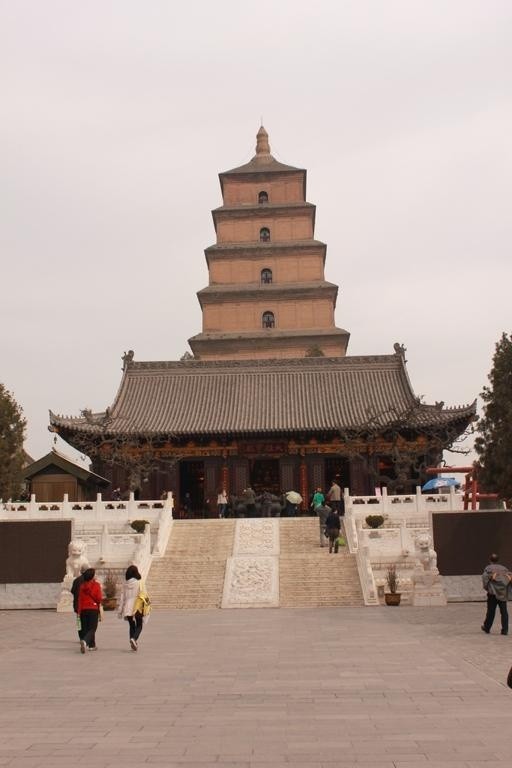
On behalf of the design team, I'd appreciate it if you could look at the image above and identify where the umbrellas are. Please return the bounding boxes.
[286,491,303,504]
[422,477,460,492]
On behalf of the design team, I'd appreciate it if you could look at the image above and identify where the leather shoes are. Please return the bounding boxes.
[481,625,489,633]
[501,629,508,635]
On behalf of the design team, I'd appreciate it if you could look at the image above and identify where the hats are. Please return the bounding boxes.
[81,564,89,574]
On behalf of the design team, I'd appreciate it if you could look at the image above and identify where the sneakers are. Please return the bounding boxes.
[79,639,97,653]
[130,637,137,651]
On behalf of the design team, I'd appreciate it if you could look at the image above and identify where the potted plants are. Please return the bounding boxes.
[101,570,117,611]
[384,566,402,606]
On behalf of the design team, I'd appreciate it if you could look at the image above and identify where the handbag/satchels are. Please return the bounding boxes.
[336,536,346,544]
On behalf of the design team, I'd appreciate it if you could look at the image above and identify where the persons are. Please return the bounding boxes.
[310,480,344,553]
[110,488,124,510]
[159,488,194,519]
[117,564,151,650]
[70,562,104,653]
[216,484,297,518]
[481,553,512,636]
